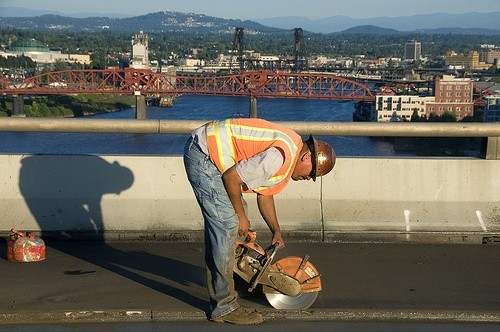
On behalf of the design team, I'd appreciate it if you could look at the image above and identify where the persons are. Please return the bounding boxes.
[183,118,337,325]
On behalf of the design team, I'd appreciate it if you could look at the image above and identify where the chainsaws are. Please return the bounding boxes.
[231,230,322,311]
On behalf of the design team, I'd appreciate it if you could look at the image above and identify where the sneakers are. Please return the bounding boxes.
[211,306,264,325]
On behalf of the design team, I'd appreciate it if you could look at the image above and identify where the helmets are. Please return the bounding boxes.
[309,133,336,182]
